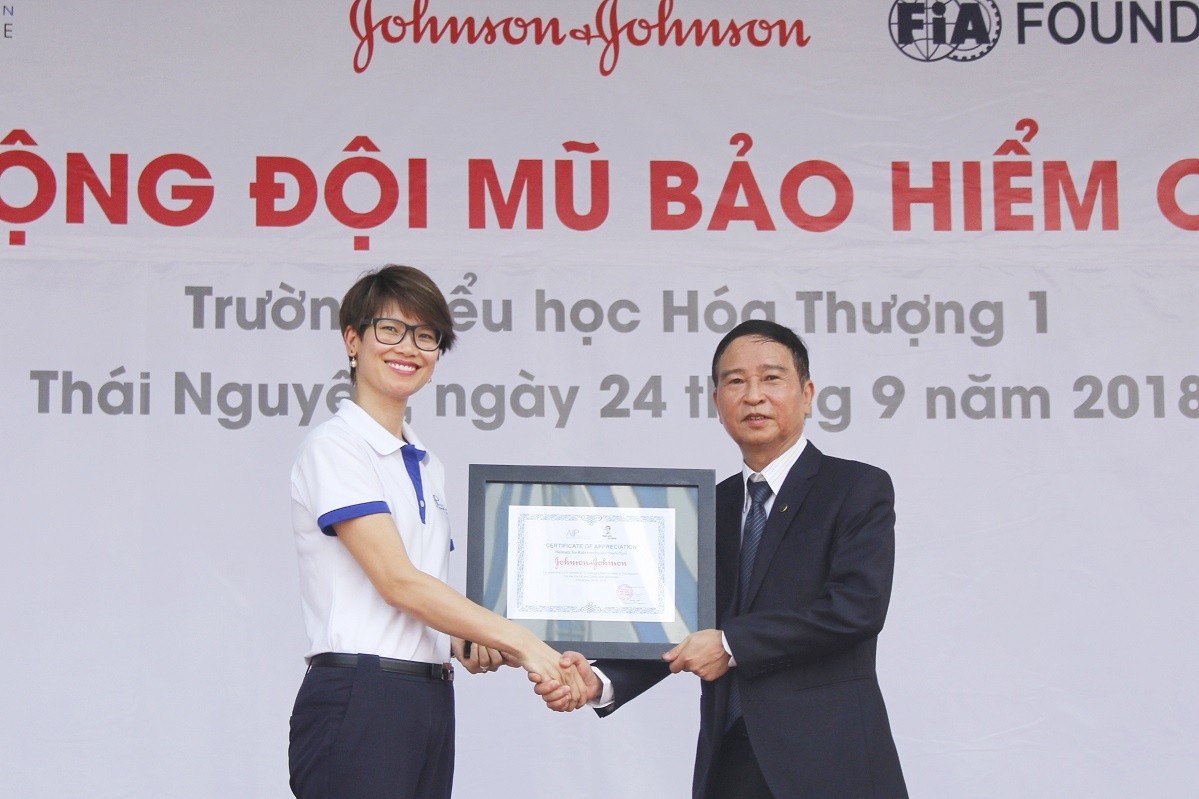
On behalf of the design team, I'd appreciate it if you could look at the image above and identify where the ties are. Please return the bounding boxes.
[722,477,773,737]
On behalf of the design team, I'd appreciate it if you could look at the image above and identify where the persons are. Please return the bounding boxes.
[288,265,592,799]
[527,319,910,799]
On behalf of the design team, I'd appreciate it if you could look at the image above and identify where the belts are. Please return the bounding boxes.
[312,652,454,683]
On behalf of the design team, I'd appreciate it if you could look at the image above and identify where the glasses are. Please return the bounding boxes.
[360,317,445,351]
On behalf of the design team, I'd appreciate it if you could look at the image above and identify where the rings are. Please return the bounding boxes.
[480,662,489,672]
